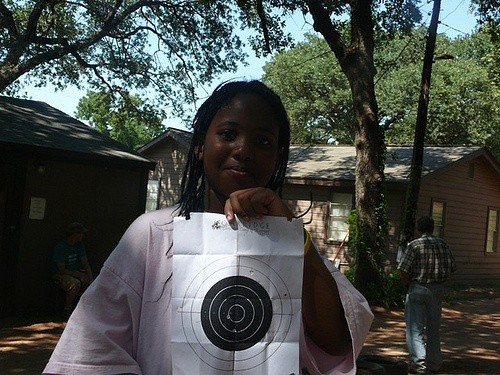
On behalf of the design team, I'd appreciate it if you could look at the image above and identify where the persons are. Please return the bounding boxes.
[399,216,456,375]
[50,223,94,322]
[40,80,376,375]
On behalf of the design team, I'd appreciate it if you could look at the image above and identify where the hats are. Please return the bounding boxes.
[65,222,88,233]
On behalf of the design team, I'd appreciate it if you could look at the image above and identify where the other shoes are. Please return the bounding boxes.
[63,306,73,323]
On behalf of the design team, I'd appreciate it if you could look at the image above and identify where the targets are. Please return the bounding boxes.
[181,255,293,372]
